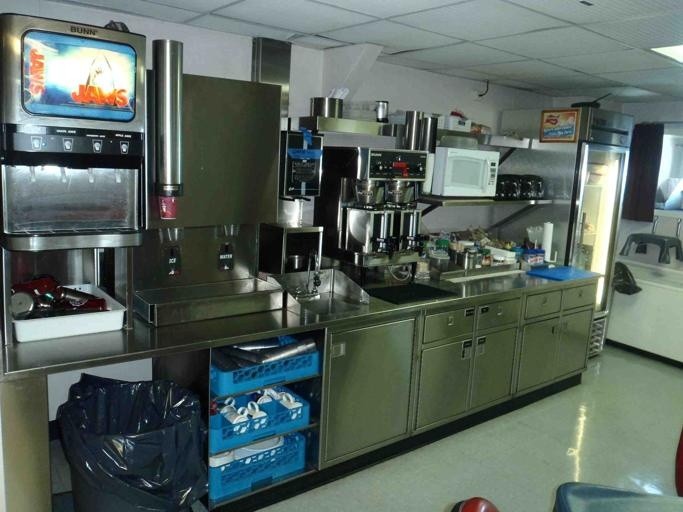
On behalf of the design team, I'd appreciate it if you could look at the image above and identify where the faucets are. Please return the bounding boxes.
[376,235,396,259]
[406,231,430,256]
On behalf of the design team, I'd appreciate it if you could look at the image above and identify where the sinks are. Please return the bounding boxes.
[363,282,458,305]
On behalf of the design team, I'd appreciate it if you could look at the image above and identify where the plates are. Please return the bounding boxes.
[209,436,285,469]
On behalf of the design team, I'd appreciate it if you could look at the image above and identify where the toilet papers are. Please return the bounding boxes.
[542,222,553,259]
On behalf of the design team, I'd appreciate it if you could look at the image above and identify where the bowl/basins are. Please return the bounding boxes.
[311,96,343,118]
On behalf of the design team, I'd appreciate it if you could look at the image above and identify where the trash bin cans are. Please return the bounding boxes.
[60,381,201,512]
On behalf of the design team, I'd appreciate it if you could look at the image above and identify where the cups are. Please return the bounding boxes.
[221,386,304,434]
[376,100,389,122]
[159,196,178,221]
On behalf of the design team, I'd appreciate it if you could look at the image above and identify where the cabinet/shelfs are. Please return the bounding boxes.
[415,296,521,429]
[516,283,597,390]
[325,319,415,462]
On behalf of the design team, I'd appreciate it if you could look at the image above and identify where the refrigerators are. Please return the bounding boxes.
[492,107,635,359]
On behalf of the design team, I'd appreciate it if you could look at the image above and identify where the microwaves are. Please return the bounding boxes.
[430,146,500,198]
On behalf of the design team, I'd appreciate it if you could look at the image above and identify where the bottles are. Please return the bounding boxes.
[505,241,545,267]
[40,286,107,314]
[450,232,493,271]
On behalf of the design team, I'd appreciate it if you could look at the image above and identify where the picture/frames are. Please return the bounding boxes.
[539,107,582,144]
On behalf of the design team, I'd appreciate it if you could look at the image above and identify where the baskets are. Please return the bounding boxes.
[208,337,321,504]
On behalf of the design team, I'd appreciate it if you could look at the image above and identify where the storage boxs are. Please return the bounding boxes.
[11,283,127,342]
[16,331,124,368]
[552,482,662,512]
